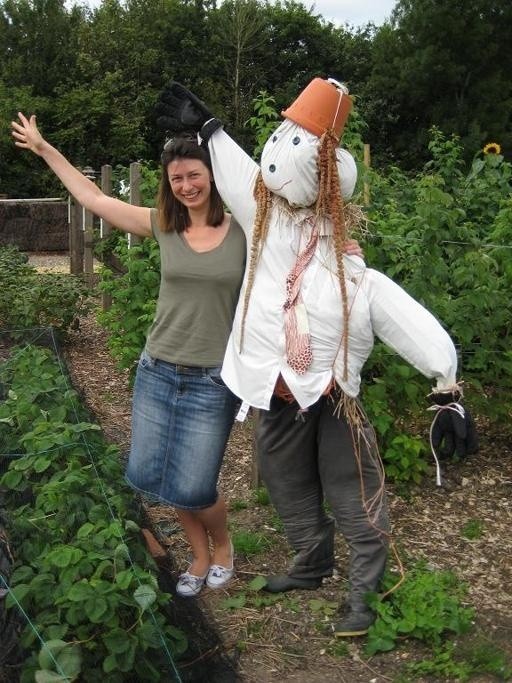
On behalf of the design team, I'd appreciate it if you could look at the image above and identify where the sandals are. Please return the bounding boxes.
[175,538,234,597]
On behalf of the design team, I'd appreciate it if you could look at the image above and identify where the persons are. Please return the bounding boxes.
[155,75,481,638]
[10,109,247,598]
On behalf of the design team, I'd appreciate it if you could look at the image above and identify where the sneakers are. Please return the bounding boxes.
[261,572,321,593]
[334,608,376,637]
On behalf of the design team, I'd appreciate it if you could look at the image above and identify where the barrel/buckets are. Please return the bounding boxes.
[279,77,355,150]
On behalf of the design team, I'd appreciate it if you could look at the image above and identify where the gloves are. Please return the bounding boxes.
[424,391,477,460]
[155,80,224,147]
